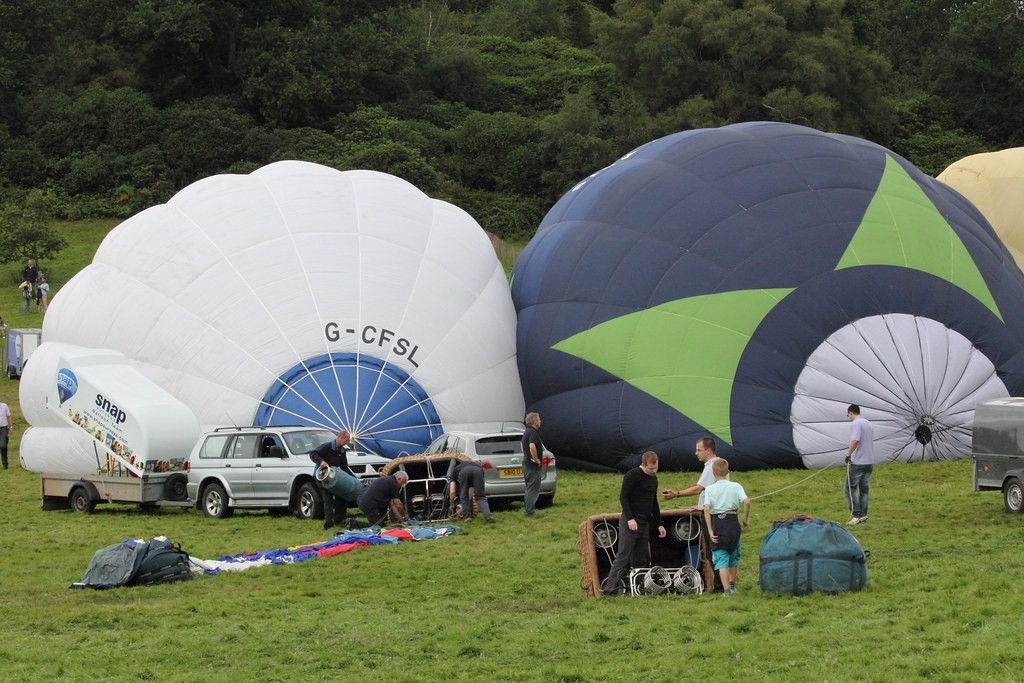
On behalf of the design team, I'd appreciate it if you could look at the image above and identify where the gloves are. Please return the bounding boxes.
[845,455,851,464]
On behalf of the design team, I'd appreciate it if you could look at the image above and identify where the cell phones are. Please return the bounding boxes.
[662,491,668,494]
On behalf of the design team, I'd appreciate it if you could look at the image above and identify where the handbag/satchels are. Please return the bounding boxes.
[138,542,189,574]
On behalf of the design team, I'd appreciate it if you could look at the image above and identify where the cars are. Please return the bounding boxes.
[424,427,557,509]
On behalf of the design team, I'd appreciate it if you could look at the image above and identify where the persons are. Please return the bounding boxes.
[520,413,543,518]
[601,450,667,596]
[662,436,730,594]
[0,316,7,372]
[152,459,190,472]
[0,402,14,470]
[703,458,751,598]
[310,430,357,531]
[449,460,496,523]
[345,470,410,531]
[845,404,874,526]
[18,259,49,315]
[67,408,144,473]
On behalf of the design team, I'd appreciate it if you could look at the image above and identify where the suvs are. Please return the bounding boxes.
[186,424,395,518]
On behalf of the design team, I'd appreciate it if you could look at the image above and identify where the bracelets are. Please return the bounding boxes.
[677,491,681,498]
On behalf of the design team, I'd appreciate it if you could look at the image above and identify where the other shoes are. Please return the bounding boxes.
[346,517,356,531]
[485,515,496,523]
[846,516,868,525]
[722,587,736,596]
